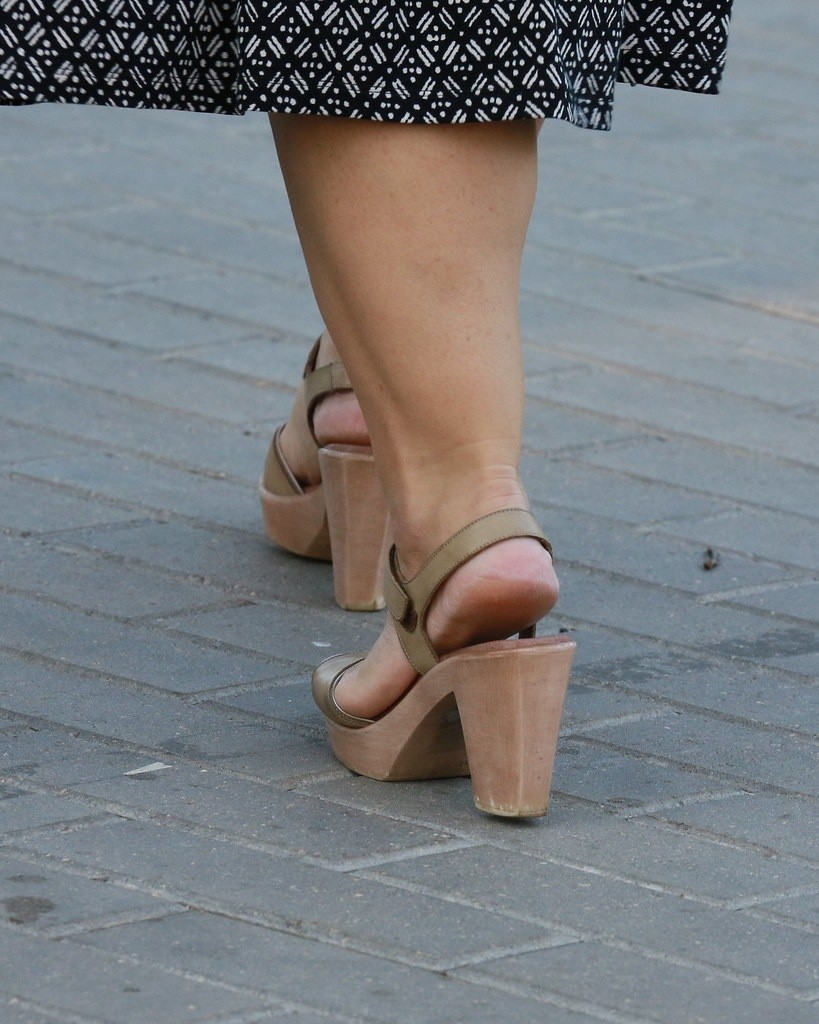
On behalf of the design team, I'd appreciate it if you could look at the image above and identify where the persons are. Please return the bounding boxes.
[0,0,738,817]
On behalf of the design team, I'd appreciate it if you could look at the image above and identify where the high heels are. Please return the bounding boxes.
[259,333,393,611]
[309,507,576,818]
